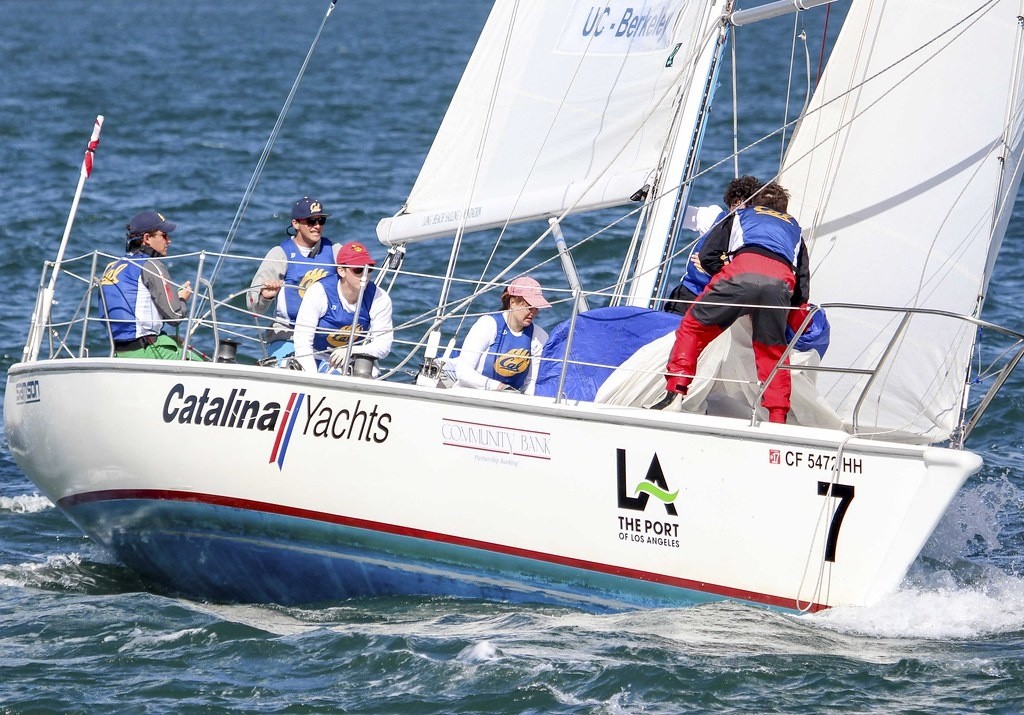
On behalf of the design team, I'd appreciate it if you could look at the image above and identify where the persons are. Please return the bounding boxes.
[453,276,552,397]
[647,183,808,424]
[246,198,342,368]
[97,211,211,363]
[294,242,394,378]
[631,175,761,318]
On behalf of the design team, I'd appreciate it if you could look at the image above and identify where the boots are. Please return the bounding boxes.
[643,389,683,412]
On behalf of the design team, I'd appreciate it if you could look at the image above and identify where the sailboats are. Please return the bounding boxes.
[0,0,1024,615]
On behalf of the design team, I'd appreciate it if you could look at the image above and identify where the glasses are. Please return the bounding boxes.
[301,216,326,226]
[152,233,166,238]
[348,267,373,274]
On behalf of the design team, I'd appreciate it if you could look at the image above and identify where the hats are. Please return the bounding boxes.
[128,209,176,233]
[292,196,331,220]
[508,276,552,309]
[337,241,376,266]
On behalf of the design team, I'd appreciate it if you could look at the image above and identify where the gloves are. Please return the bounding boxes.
[327,344,352,365]
